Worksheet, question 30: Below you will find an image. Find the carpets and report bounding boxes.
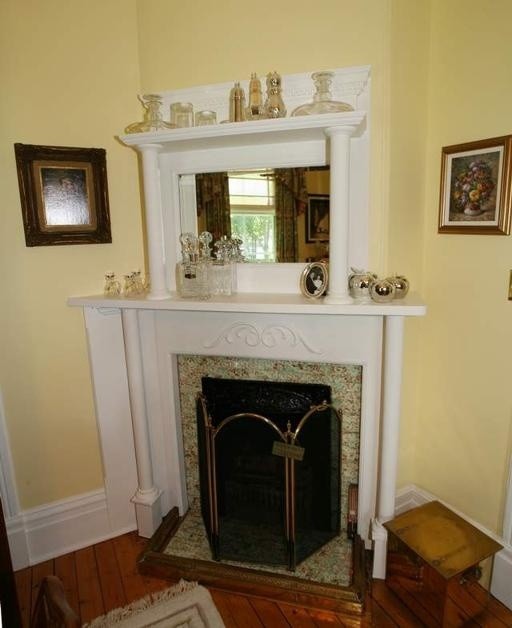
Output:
[71,577,227,627]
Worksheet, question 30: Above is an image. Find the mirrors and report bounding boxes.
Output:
[174,163,332,267]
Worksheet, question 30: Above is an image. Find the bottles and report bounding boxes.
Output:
[291,71,355,116]
[125,93,176,134]
[176,231,235,301]
[228,70,288,121]
[104,271,144,298]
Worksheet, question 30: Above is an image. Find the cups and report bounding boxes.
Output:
[170,102,217,128]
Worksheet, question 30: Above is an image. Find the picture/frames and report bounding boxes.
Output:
[298,257,331,300]
[12,142,114,248]
[434,134,512,237]
[303,191,330,246]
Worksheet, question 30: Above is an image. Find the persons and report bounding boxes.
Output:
[307,271,324,295]
